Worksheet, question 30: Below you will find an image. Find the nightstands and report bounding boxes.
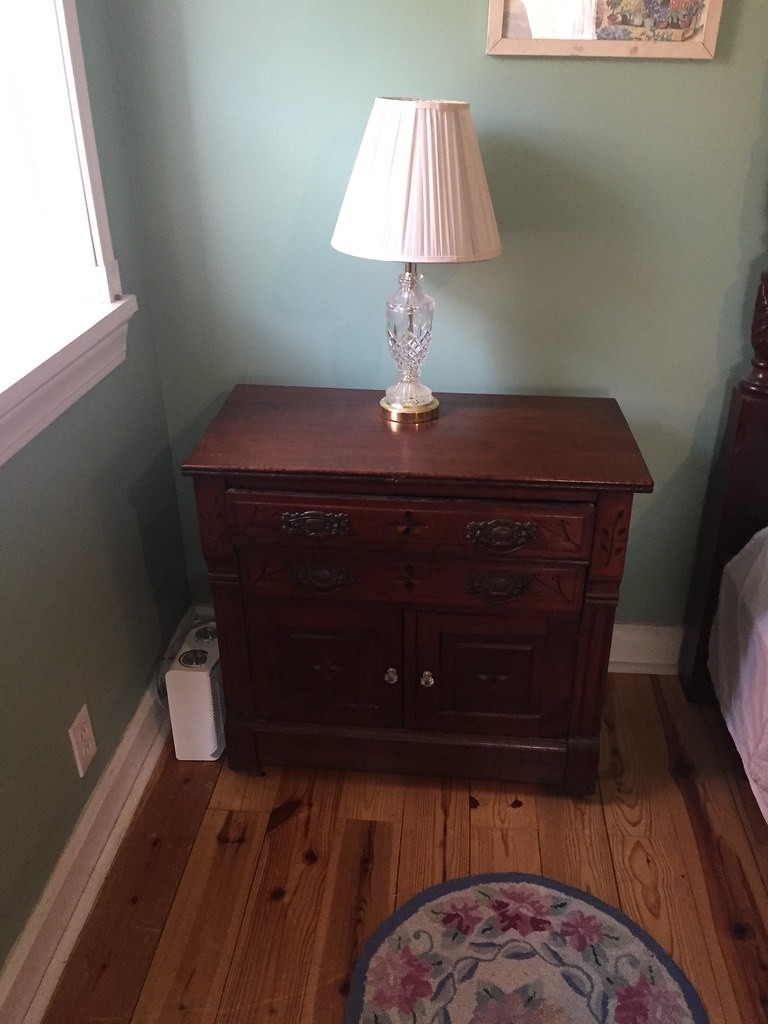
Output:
[184,388,656,801]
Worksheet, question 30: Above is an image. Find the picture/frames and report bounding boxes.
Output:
[485,0,723,60]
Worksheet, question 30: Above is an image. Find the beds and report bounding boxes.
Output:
[675,269,768,824]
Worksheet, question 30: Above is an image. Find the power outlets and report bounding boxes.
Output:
[68,702,97,778]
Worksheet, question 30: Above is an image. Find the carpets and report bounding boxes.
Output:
[344,872,710,1024]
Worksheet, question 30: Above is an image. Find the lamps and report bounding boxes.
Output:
[331,96,501,426]
